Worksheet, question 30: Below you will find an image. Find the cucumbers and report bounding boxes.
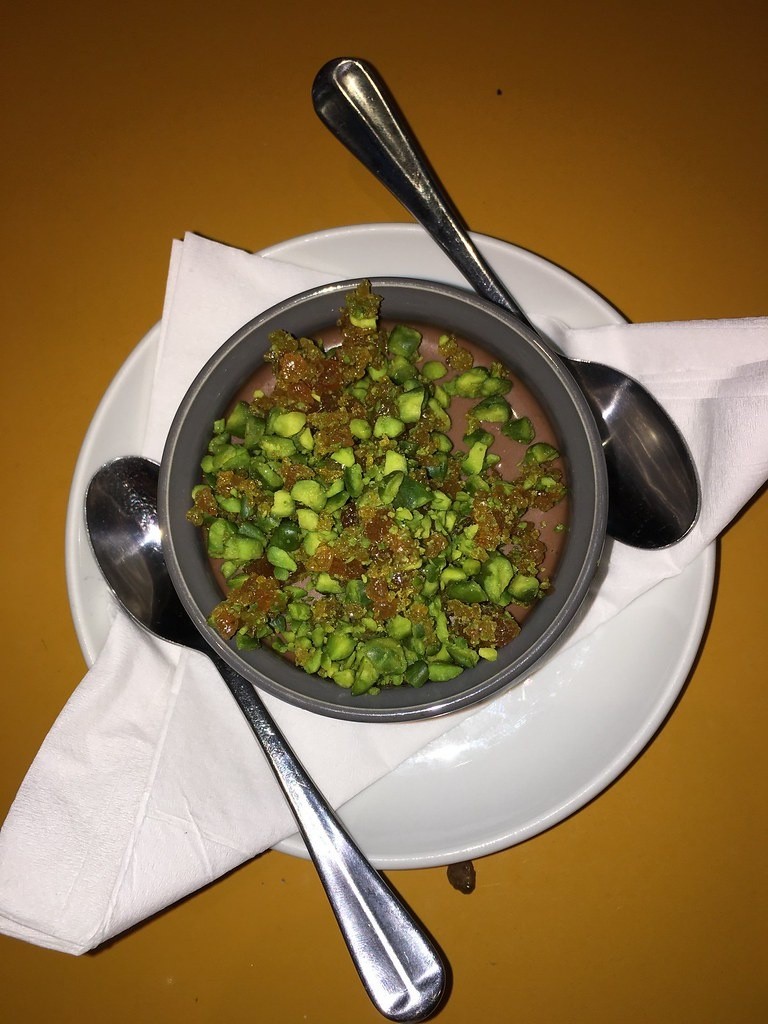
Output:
[191,308,560,692]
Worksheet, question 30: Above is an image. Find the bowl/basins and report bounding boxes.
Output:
[157,275,611,726]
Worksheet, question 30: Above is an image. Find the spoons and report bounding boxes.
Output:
[310,54,702,552]
[85,453,452,1024]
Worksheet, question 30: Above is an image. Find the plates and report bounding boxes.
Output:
[63,223,720,871]
